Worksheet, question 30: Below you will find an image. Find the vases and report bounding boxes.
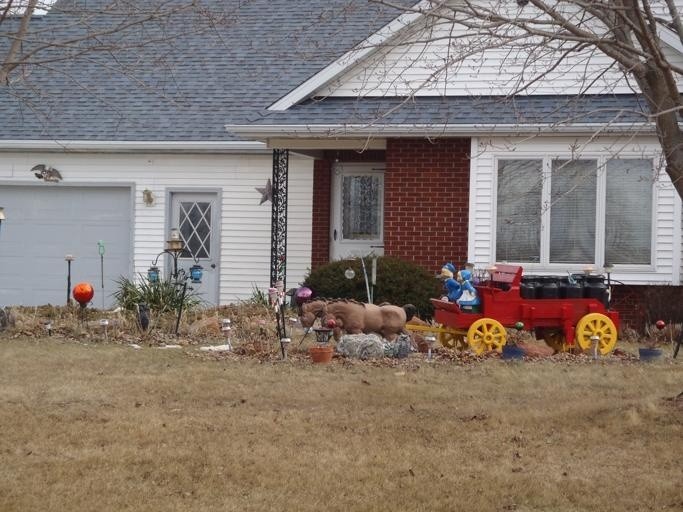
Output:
[310,347,332,364]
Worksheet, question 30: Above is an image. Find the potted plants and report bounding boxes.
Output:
[638,320,666,365]
